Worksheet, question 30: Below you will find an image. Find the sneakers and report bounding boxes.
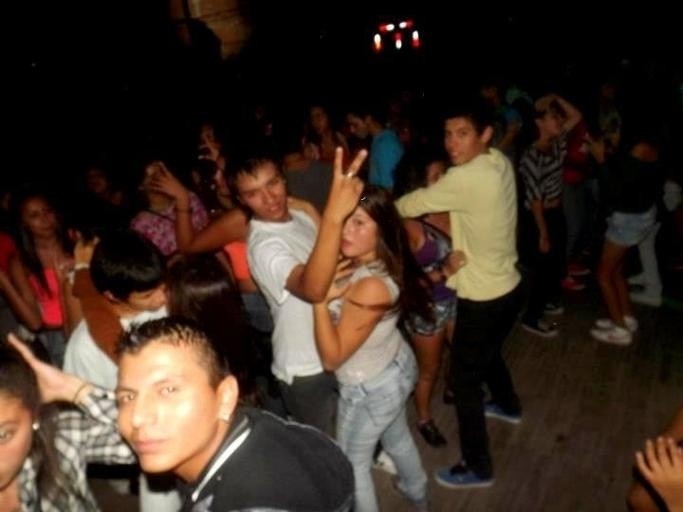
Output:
[432,461,497,492]
[441,388,466,409]
[416,419,450,446]
[483,397,527,427]
[516,268,668,346]
[372,444,432,512]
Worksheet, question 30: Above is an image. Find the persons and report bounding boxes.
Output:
[2,82,682,512]
